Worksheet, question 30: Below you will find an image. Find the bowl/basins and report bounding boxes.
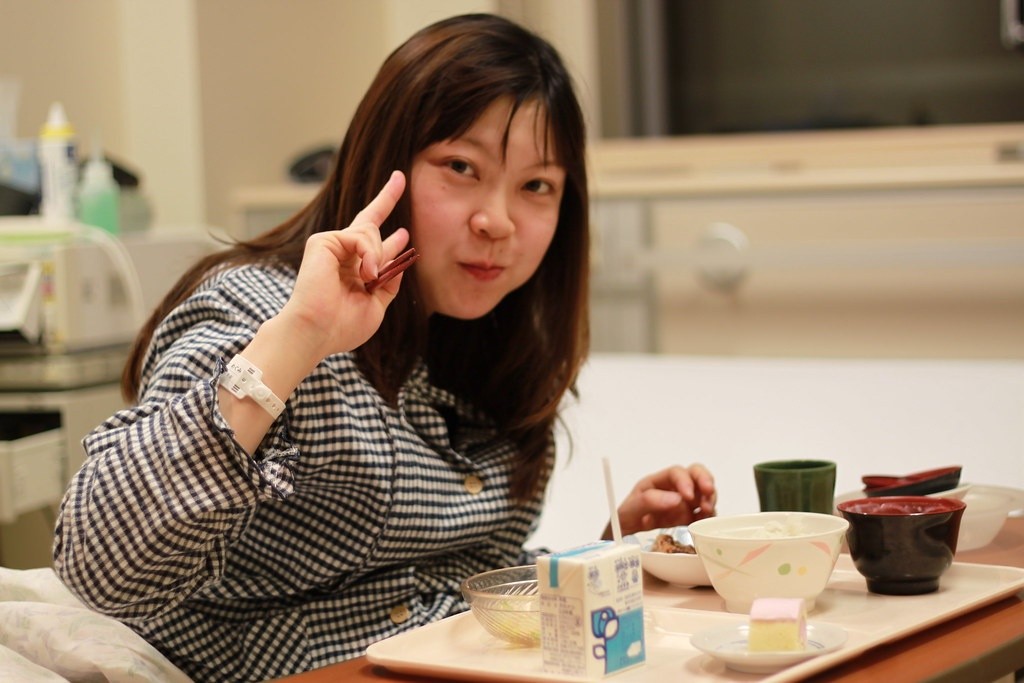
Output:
[838,466,1024,596]
[462,565,542,648]
[623,526,714,586]
[687,511,850,614]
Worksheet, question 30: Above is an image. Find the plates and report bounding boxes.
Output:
[691,623,848,673]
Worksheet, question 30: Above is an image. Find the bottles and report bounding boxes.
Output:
[37,104,77,229]
[78,150,120,237]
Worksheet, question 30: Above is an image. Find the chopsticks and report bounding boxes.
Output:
[365,248,419,293]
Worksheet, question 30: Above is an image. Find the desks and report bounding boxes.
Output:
[267,484,1024,683]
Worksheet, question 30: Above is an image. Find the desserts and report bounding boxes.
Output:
[746,596,807,652]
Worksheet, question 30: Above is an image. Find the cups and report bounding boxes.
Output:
[754,459,837,515]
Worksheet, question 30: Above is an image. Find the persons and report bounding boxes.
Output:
[53,15,717,683]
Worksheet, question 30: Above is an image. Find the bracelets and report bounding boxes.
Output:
[218,354,286,420]
